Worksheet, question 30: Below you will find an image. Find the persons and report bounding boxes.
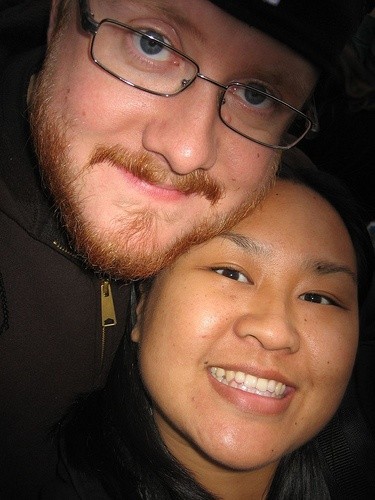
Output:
[0,0,375,500]
[46,146,375,500]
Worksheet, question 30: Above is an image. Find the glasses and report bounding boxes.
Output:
[74,0,320,152]
